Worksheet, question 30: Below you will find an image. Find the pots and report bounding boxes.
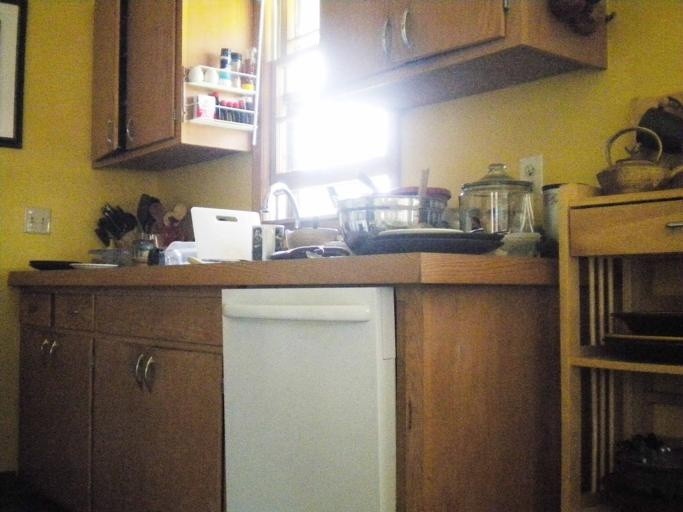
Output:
[612,431,683,502]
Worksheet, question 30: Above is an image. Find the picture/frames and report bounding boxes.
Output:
[0,0,29,149]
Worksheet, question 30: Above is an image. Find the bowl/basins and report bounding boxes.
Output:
[337,195,451,255]
[286,227,338,250]
[497,230,545,258]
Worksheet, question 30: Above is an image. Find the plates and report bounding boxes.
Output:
[269,246,349,257]
[29,260,119,270]
[610,310,683,336]
[601,332,683,366]
[349,227,505,255]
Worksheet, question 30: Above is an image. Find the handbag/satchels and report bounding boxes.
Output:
[636,105,683,152]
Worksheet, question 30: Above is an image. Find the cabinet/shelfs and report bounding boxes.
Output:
[17,287,94,512]
[556,183,683,510]
[93,290,221,512]
[90,0,261,170]
[320,1,609,110]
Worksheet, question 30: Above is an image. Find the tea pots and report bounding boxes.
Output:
[595,126,683,196]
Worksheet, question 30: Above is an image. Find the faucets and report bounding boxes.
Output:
[260,181,303,229]
